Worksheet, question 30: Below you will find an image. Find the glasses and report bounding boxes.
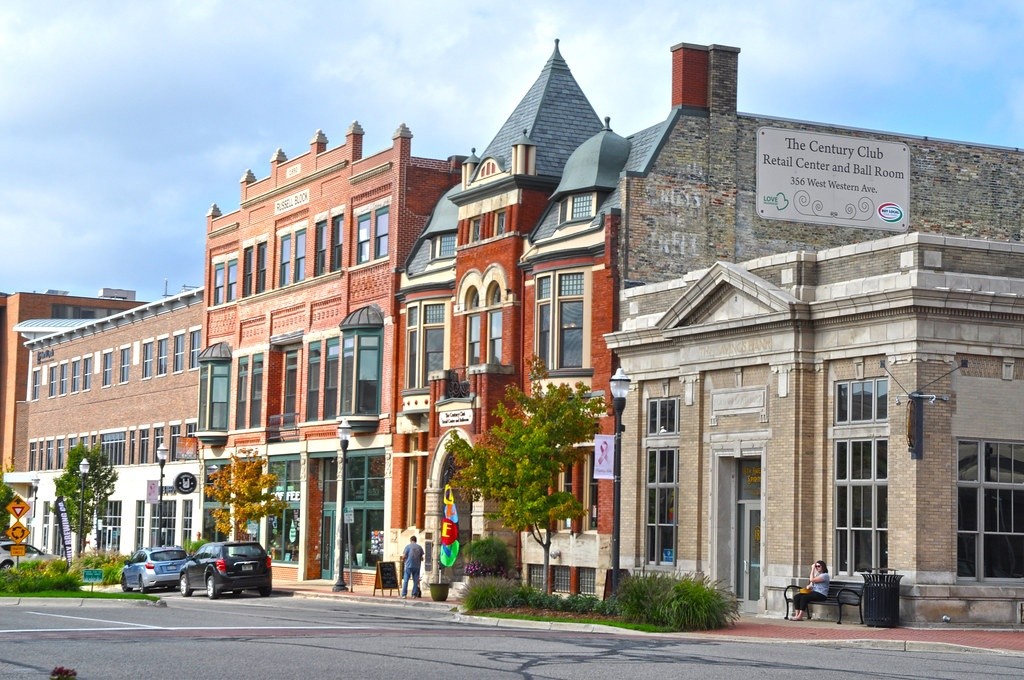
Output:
[815,565,821,567]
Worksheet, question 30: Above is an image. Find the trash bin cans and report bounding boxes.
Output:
[860,568,905,627]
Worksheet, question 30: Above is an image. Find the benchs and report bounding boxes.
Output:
[783,580,865,625]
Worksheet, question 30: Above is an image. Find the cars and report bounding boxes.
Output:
[0,542,57,571]
[119,546,192,593]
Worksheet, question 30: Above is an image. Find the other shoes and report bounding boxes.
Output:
[792,617,803,621]
[789,616,796,620]
[402,596,406,598]
[412,595,416,598]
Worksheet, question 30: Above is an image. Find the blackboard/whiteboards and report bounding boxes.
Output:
[378,562,399,589]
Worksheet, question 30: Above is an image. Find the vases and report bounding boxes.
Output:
[429,582,449,601]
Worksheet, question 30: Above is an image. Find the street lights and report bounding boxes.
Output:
[607,367,631,592]
[156,443,169,546]
[332,418,352,594]
[31,473,41,546]
[77,457,91,558]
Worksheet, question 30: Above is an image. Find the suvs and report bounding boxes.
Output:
[179,539,273,600]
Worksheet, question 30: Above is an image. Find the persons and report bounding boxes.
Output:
[789,560,829,621]
[400,536,424,599]
[197,532,201,540]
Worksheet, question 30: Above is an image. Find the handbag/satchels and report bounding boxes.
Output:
[799,588,812,594]
